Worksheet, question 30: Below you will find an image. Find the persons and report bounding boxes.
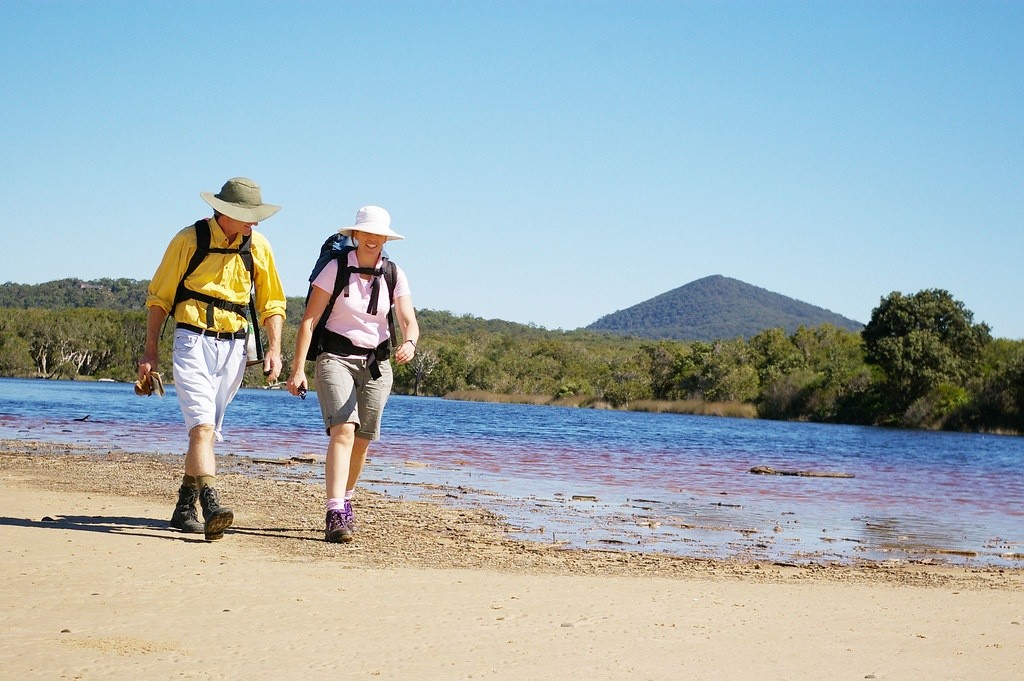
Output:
[286,205,420,541]
[136,176,287,541]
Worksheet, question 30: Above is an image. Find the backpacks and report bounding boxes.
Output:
[305,232,398,348]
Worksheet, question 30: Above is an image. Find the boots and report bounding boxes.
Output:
[198,483,234,541]
[168,484,205,533]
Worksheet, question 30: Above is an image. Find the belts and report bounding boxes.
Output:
[176,321,247,339]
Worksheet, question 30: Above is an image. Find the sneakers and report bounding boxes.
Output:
[324,508,353,543]
[343,499,359,531]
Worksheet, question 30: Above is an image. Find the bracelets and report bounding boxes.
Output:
[404,340,417,350]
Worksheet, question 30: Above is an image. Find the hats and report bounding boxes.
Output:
[338,206,405,242]
[200,177,281,223]
